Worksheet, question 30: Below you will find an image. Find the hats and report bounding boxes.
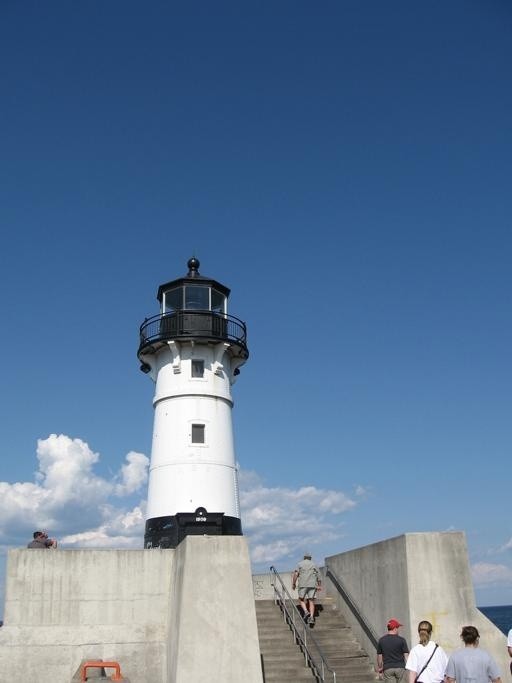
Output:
[387,619,403,627]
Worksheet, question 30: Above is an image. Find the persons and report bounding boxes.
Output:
[377,620,409,683]
[506,629,512,676]
[405,621,456,683]
[27,531,58,548]
[443,625,503,683]
[292,553,322,623]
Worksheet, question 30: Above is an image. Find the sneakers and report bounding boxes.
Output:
[302,611,311,620]
[307,616,315,623]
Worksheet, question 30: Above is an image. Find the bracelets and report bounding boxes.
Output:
[379,669,384,673]
